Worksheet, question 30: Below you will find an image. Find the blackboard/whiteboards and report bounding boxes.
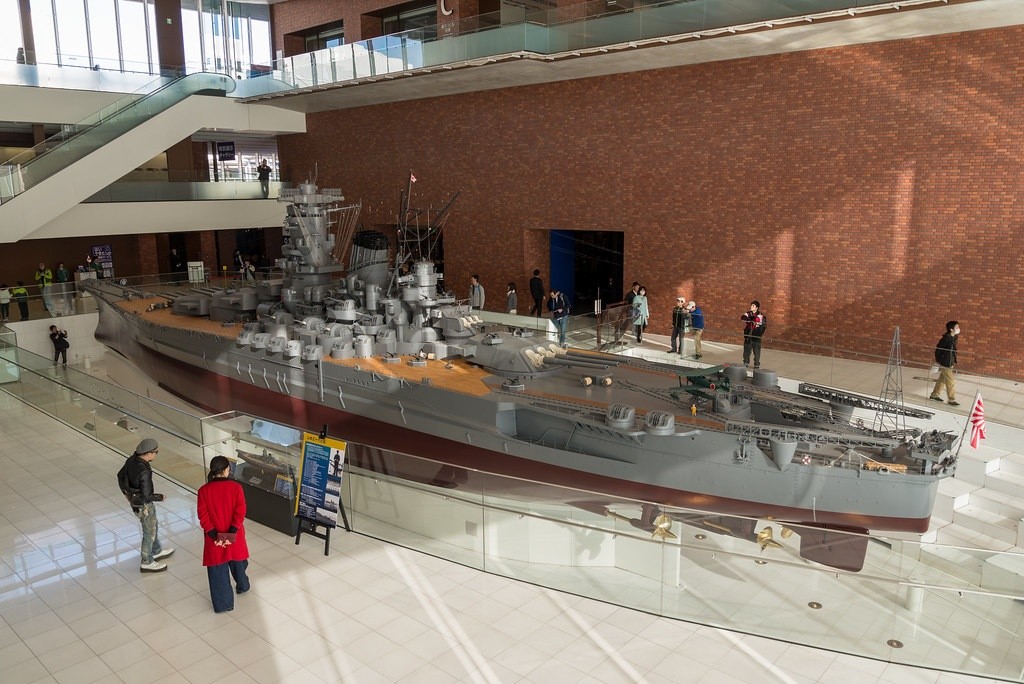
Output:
[187,261,205,283]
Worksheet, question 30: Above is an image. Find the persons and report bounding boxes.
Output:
[632,287,649,344]
[17,48,25,64]
[233,249,243,280]
[530,269,545,318]
[332,451,340,476]
[691,404,696,416]
[168,250,182,285]
[667,297,703,361]
[929,321,960,405]
[504,282,517,313]
[399,264,408,276]
[547,289,570,348]
[240,260,255,281]
[626,281,640,337]
[0,256,104,323]
[49,325,68,378]
[117,439,175,573]
[467,275,485,310]
[741,301,766,369]
[198,456,250,614]
[258,159,271,198]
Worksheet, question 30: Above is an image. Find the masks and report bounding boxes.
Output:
[954,328,960,334]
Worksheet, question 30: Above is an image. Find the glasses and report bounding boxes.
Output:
[149,450,159,454]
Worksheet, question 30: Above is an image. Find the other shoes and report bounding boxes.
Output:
[755,363,760,367]
[677,350,681,355]
[667,349,676,353]
[743,363,749,366]
[948,401,959,405]
[930,395,943,401]
[694,354,701,360]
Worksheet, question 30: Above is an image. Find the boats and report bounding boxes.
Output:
[77,159,974,577]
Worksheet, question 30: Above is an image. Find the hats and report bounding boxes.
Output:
[632,281,641,287]
[686,301,696,309]
[136,438,159,454]
[676,297,685,303]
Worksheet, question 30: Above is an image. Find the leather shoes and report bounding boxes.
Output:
[153,548,174,561]
[140,560,167,572]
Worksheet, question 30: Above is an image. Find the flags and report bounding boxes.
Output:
[969,397,986,449]
